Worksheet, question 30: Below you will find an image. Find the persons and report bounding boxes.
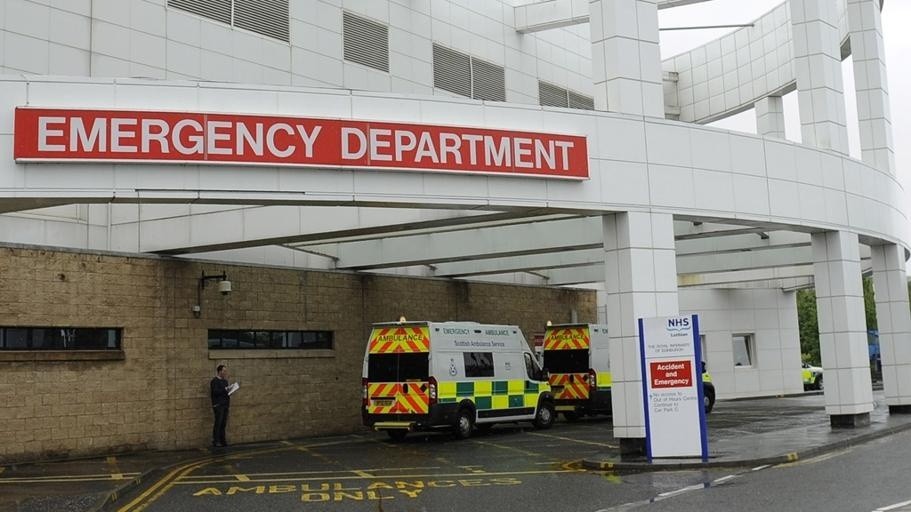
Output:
[211,365,231,447]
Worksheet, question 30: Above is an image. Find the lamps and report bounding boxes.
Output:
[201,267,231,296]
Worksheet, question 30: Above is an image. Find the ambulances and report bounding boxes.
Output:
[542,322,716,420]
[362,316,556,442]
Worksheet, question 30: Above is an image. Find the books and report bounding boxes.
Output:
[228,383,240,396]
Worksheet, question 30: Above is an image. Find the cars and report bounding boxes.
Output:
[802,362,824,390]
